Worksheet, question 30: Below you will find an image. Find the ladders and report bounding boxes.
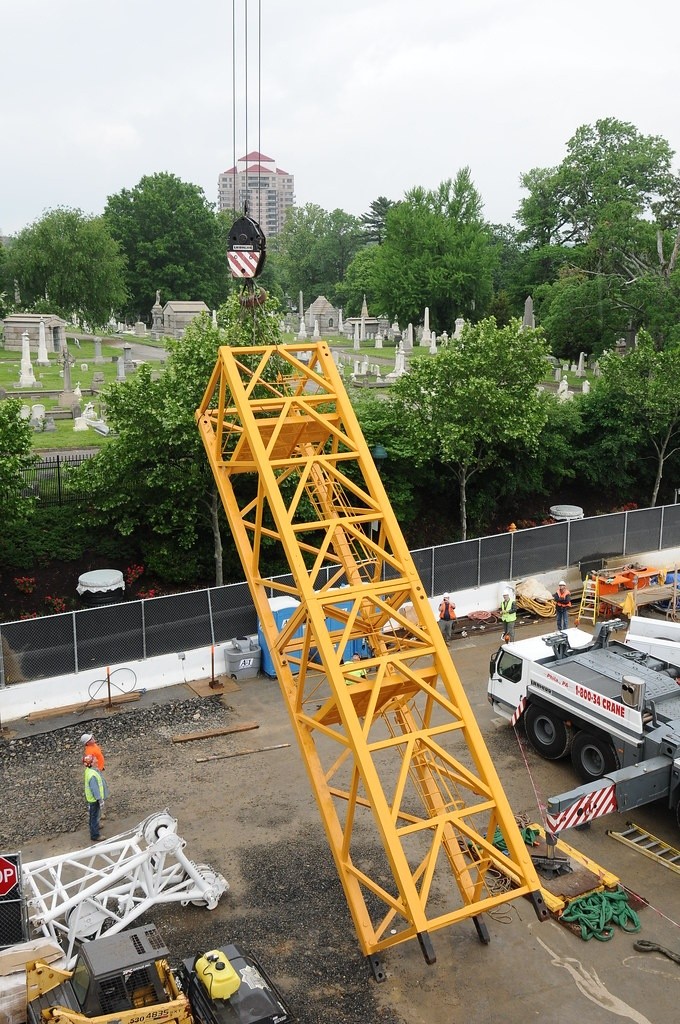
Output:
[576,575,599,627]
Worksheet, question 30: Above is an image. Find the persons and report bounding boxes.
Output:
[344,652,366,687]
[555,581,571,630]
[81,734,105,772]
[84,755,105,841]
[438,592,455,647]
[499,592,517,643]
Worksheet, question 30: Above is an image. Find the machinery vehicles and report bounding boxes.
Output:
[24,922,292,1024]
[485,619,680,825]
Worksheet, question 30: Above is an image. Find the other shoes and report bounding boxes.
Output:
[446,641,450,646]
[100,812,107,820]
[93,835,106,841]
[99,823,104,828]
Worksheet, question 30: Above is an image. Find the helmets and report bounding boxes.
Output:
[81,734,92,745]
[559,581,566,585]
[82,754,93,767]
[443,593,449,597]
[503,590,509,595]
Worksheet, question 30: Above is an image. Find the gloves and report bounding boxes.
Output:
[500,610,507,615]
[98,799,105,810]
